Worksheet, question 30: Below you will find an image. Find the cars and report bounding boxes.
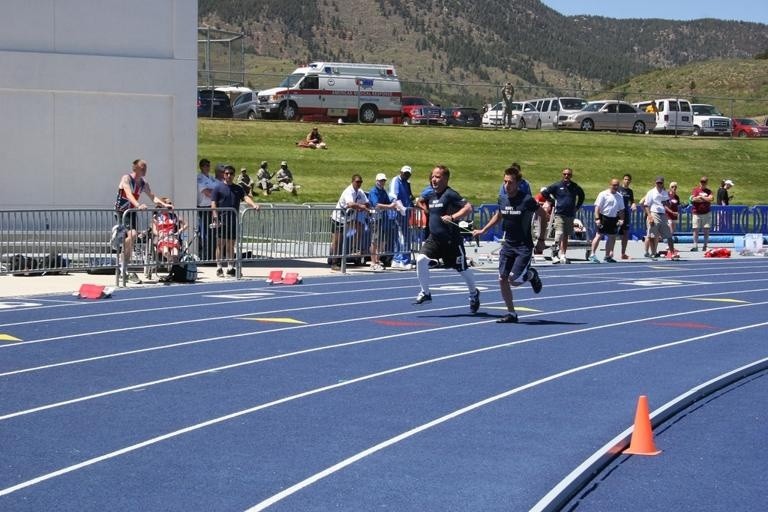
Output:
[550,100,658,134]
[729,118,768,139]
[479,101,543,130]
[231,89,263,120]
[440,107,482,128]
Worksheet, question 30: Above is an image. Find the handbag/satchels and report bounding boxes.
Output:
[87,257,117,274]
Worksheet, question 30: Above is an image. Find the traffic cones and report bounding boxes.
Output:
[620,394,667,458]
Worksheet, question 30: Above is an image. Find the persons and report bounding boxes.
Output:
[471,167,547,323]
[151,198,188,263]
[197,159,225,265]
[500,162,532,195]
[326,165,440,276]
[587,174,680,264]
[501,82,514,130]
[306,127,322,148]
[690,176,714,251]
[645,101,659,134]
[210,165,260,276]
[276,160,297,194]
[237,168,254,198]
[534,187,555,221]
[717,180,735,231]
[410,165,480,313]
[546,168,585,264]
[256,161,279,195]
[114,159,175,284]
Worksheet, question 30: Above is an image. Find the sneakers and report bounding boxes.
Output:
[496,313,518,323]
[370,262,385,271]
[604,256,616,262]
[530,268,542,294]
[552,256,560,263]
[226,268,242,277]
[416,291,432,301]
[119,273,128,282]
[588,255,600,263]
[690,247,698,252]
[391,261,415,270]
[331,263,340,270]
[469,288,479,313]
[217,268,224,277]
[128,273,142,284]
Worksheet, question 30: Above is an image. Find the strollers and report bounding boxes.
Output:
[140,204,187,278]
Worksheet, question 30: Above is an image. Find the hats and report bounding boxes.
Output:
[241,168,246,171]
[281,160,288,166]
[656,176,664,183]
[400,166,412,174]
[725,180,734,186]
[376,173,387,181]
[700,176,708,182]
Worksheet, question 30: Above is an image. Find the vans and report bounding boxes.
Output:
[629,98,695,136]
[528,97,589,132]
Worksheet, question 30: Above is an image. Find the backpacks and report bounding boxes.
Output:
[174,251,197,281]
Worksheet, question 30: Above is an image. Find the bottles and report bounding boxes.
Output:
[209,222,223,229]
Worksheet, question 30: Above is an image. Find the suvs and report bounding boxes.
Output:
[691,103,733,137]
[197,88,233,119]
[394,96,442,126]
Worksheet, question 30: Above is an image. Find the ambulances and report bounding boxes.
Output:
[255,61,403,124]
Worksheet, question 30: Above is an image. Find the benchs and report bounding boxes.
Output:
[494,233,592,260]
